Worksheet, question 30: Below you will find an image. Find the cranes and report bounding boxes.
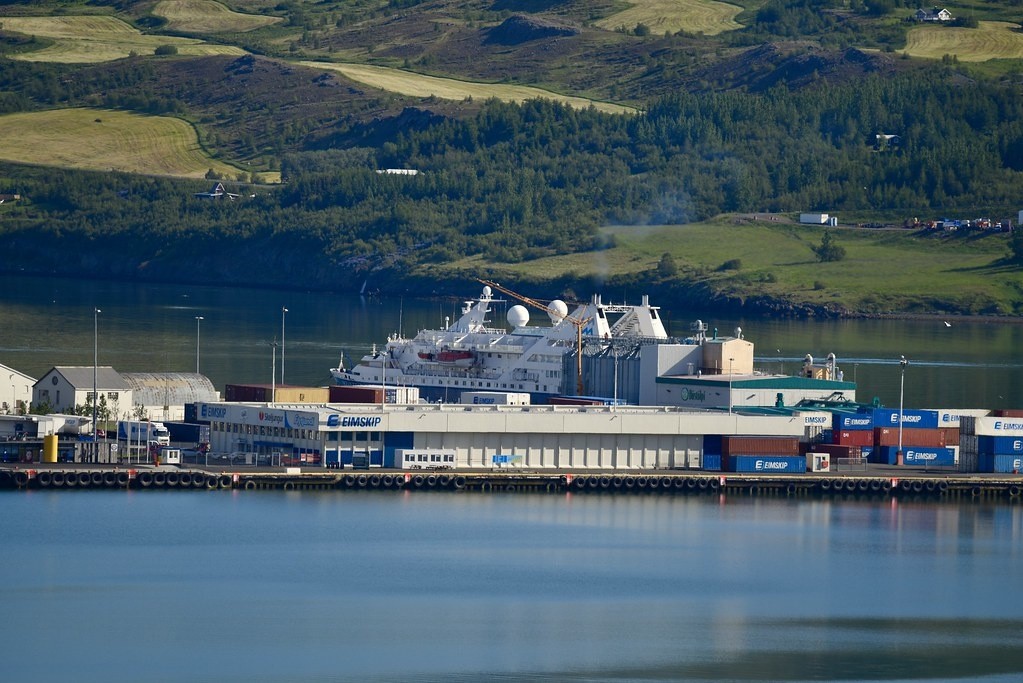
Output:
[473,276,594,393]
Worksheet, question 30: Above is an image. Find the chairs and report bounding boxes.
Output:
[409,464,452,471]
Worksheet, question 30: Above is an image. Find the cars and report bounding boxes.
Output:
[275,457,301,466]
[211,452,270,462]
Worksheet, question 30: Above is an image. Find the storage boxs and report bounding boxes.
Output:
[703,405,1023,476]
[225,382,531,405]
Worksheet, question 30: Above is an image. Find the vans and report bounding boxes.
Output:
[180,448,202,457]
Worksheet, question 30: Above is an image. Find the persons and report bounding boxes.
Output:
[22,450,33,463]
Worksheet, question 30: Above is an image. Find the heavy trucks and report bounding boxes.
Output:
[118,421,169,446]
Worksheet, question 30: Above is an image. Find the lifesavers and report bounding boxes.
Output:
[219,476,232,488]
[78,473,91,487]
[205,477,218,488]
[15,473,29,486]
[117,473,130,486]
[154,473,166,486]
[193,474,205,487]
[140,472,153,487]
[179,474,192,486]
[52,472,64,486]
[283,481,295,491]
[166,473,179,486]
[104,473,117,486]
[357,475,1021,498]
[92,473,103,485]
[244,480,256,490]
[346,475,355,487]
[65,473,78,486]
[38,473,51,486]
[4,473,12,484]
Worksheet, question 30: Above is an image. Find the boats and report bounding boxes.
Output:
[327,286,671,403]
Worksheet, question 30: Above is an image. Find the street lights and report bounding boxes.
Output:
[897,355,908,465]
[195,316,204,373]
[93,306,101,441]
[283,305,289,384]
